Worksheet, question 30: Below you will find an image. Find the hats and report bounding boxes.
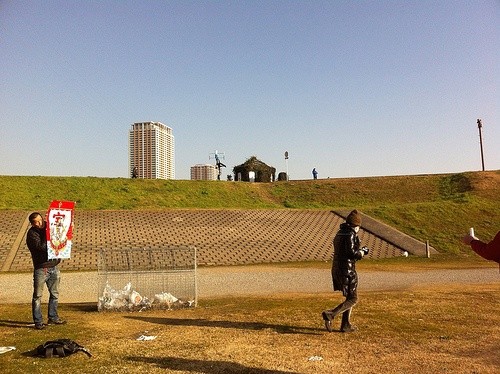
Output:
[346,209,362,226]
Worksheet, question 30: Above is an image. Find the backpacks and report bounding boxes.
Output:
[38,339,79,358]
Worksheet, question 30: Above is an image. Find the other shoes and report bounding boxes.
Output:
[35,322,45,330]
[322,310,334,332]
[340,322,355,332]
[47,318,67,325]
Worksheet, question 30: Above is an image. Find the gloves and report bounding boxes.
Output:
[362,246,369,255]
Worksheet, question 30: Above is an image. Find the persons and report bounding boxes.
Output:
[132,168,138,178]
[322,210,369,333]
[26,212,74,329]
[464,231,500,268]
[313,168,318,179]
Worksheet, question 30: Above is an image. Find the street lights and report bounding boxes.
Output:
[477,118,486,171]
